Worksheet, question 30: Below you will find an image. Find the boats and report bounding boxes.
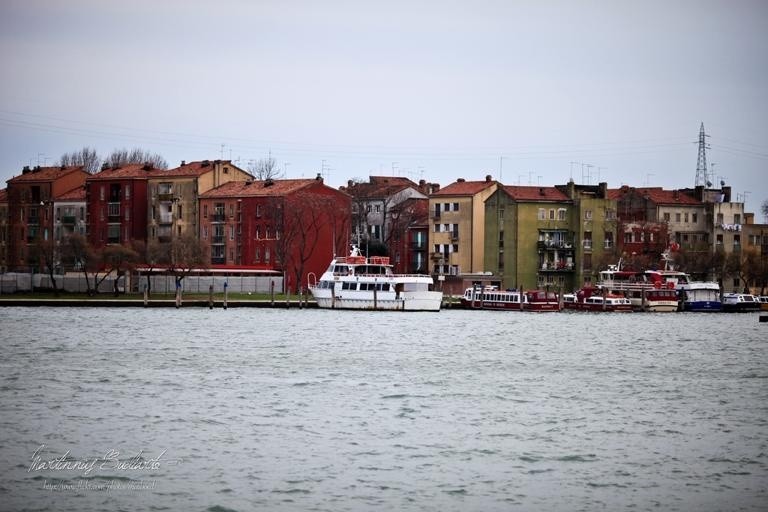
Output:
[307,256,443,310]
